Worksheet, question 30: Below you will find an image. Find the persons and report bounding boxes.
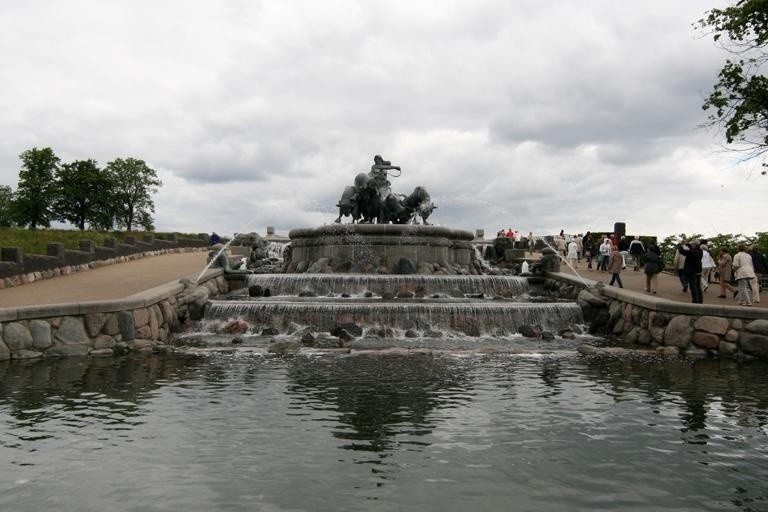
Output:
[209,232,220,247]
[496,228,521,249]
[555,230,767,306]
[350,155,392,211]
[527,232,537,255]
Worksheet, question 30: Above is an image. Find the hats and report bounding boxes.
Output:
[634,234,640,239]
[689,239,700,246]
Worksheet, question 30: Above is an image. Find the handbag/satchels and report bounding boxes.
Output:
[645,261,664,274]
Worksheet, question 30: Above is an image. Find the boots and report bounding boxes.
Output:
[588,266,639,272]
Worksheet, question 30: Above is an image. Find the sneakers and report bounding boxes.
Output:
[703,284,708,291]
[733,290,739,298]
[683,285,688,293]
[644,287,651,292]
[652,289,657,294]
[716,294,727,298]
[737,299,760,306]
